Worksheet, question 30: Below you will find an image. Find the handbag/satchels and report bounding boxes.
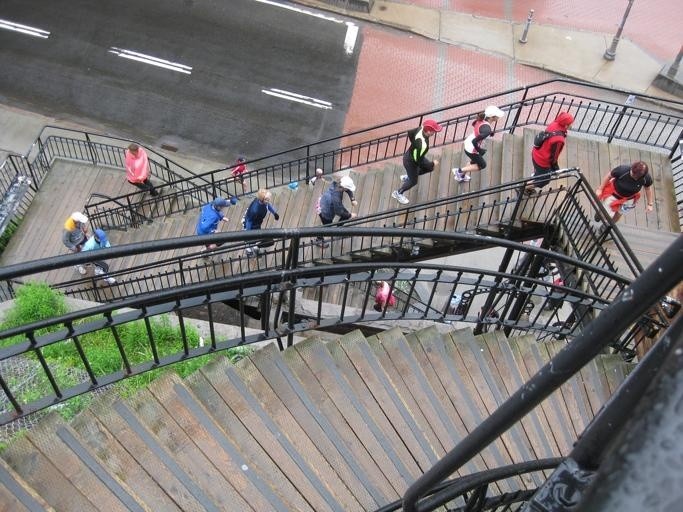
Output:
[533,131,566,149]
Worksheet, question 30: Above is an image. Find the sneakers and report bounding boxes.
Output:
[515,183,537,196]
[454,176,472,182]
[392,190,409,204]
[311,237,330,249]
[253,246,264,258]
[400,174,409,182]
[94,268,109,278]
[73,265,88,275]
[245,245,253,257]
[595,211,601,223]
[451,167,464,183]
[104,277,118,286]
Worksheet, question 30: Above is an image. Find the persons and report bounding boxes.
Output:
[516,111,574,195]
[62,212,89,252]
[594,161,653,233]
[124,144,158,196]
[313,176,358,248]
[231,158,247,184]
[391,120,442,204]
[240,189,279,254]
[197,197,229,251]
[79,229,116,284]
[634,280,683,362]
[373,281,390,312]
[451,105,505,183]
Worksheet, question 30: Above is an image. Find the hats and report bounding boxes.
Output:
[341,176,357,192]
[422,119,443,132]
[484,106,505,118]
[288,179,299,190]
[70,211,89,224]
[236,155,248,163]
[214,197,230,208]
[94,228,108,243]
